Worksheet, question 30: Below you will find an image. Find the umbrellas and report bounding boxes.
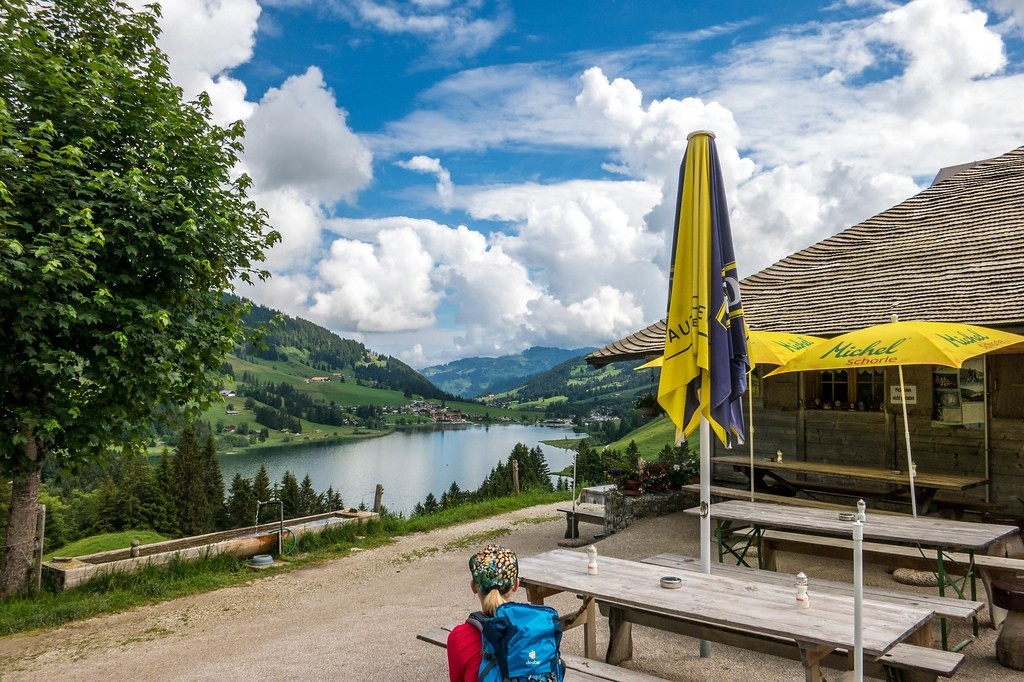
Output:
[636,322,1024,519]
[657,128,752,663]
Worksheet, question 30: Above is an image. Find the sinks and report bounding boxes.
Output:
[42,509,381,591]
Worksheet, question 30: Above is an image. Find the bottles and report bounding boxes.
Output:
[777,450,783,463]
[587,544,598,574]
[795,571,810,608]
[912,461,917,477]
[857,499,866,522]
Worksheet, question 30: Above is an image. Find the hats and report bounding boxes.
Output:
[469,544,520,596]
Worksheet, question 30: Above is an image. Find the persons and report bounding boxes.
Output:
[447,544,520,682]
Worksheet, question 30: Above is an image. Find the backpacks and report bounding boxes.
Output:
[469,601,566,682]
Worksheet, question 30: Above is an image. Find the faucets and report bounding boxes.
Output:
[256,500,267,507]
